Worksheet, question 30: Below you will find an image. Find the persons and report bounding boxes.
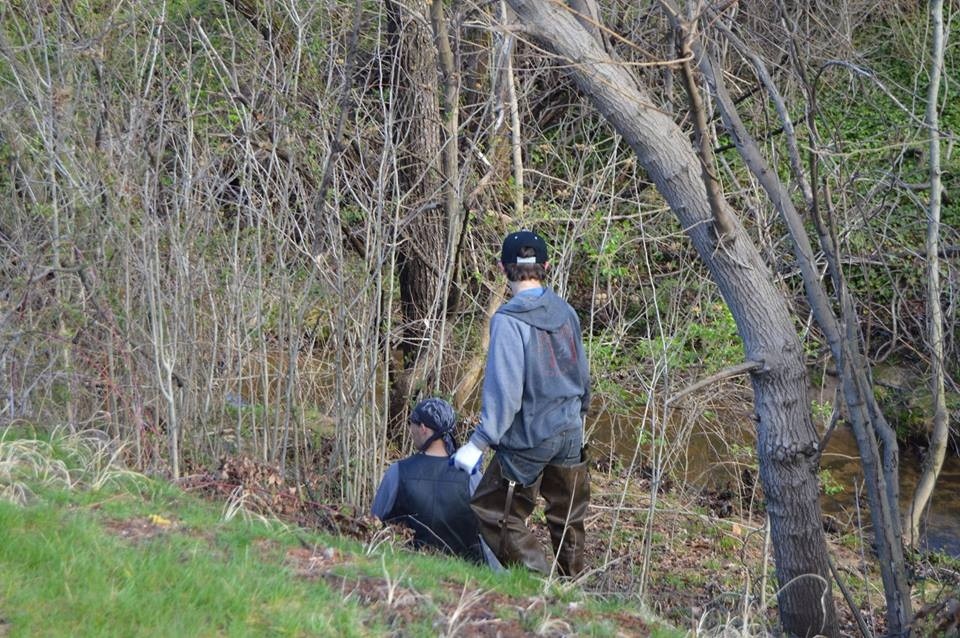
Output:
[454,232,590,586]
[371,398,503,569]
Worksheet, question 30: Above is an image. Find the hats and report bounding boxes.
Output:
[501,232,547,263]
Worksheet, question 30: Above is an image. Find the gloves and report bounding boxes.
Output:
[449,441,483,476]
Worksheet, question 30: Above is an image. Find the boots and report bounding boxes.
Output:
[473,454,548,579]
[540,448,592,583]
